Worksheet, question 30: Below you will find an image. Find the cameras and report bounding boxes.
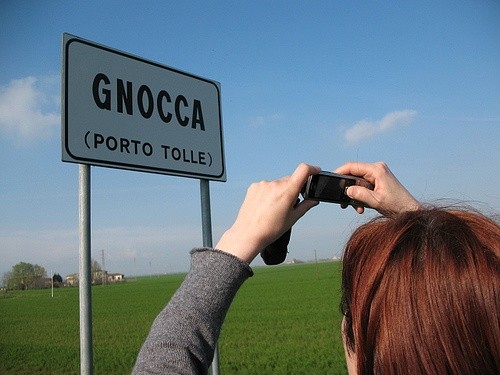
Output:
[300,170,375,208]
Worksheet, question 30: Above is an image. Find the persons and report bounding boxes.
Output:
[134,165,500,375]
